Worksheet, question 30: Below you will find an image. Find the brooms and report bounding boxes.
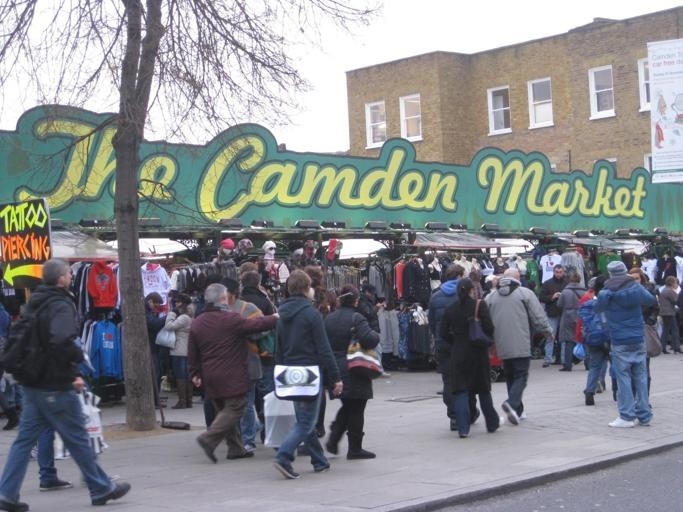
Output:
[147,336,190,429]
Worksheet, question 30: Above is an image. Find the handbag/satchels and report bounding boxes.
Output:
[273,364,320,400]
[643,322,662,356]
[155,327,176,350]
[346,335,382,380]
[467,317,494,347]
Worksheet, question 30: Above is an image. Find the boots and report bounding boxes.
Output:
[172,379,192,409]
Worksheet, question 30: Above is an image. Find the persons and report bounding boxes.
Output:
[439,278,499,438]
[216,239,307,278]
[417,256,529,285]
[484,268,555,426]
[428,264,480,431]
[145,261,392,480]
[0,258,131,512]
[37,427,74,492]
[539,259,683,429]
[0,304,24,430]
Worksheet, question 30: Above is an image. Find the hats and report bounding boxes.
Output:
[606,260,626,278]
[175,294,191,305]
[503,268,520,281]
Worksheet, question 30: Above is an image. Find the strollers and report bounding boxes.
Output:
[487,346,504,382]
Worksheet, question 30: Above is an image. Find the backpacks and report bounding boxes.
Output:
[578,302,610,346]
[0,297,76,387]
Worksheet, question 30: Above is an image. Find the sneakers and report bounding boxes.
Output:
[0,499,29,512]
[39,480,71,491]
[92,482,130,505]
[596,380,603,393]
[542,357,573,371]
[585,391,594,405]
[275,421,376,479]
[197,436,217,463]
[450,401,526,437]
[228,443,256,460]
[608,418,635,427]
